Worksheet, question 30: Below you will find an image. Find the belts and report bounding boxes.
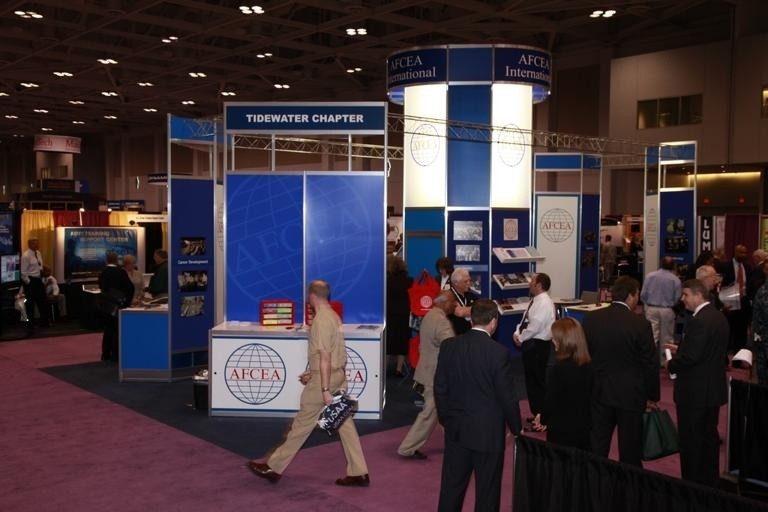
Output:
[648,304,672,308]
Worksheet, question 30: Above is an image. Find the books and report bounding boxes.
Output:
[494,245,544,315]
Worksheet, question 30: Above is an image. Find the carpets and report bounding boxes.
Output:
[34,357,525,464]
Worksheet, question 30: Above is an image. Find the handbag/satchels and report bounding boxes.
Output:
[318,392,356,436]
[640,404,681,461]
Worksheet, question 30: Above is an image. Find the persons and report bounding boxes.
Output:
[120,255,146,305]
[395,288,458,461]
[14,285,30,322]
[432,256,456,287]
[629,232,642,276]
[598,234,619,283]
[529,316,595,461]
[387,252,412,378]
[178,238,208,316]
[663,278,731,486]
[579,276,660,470]
[19,235,52,328]
[513,272,558,433]
[432,296,522,511]
[446,268,480,335]
[639,244,768,447]
[98,251,133,360]
[245,278,372,489]
[140,249,170,302]
[39,265,66,320]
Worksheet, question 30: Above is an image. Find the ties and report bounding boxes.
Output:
[35,250,41,266]
[737,264,744,297]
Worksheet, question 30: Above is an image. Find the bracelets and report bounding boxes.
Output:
[320,383,331,394]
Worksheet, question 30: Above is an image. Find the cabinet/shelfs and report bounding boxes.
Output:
[492,247,546,316]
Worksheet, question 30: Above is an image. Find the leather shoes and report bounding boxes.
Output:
[335,474,369,486]
[409,451,428,459]
[247,460,281,483]
